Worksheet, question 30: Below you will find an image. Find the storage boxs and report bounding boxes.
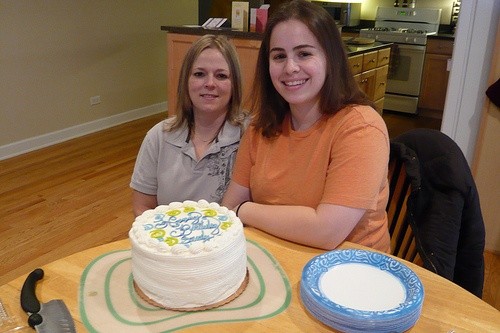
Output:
[232,2,271,31]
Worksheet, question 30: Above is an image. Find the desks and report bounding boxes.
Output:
[0,226,500,333]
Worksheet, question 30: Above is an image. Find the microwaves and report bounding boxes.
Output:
[310,1,351,26]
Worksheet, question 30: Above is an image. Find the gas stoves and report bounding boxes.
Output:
[359,5,443,43]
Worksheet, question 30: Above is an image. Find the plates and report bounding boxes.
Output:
[300,248,424,333]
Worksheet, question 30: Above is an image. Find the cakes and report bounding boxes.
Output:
[128,199,247,309]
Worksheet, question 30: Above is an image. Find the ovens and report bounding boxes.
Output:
[357,28,427,115]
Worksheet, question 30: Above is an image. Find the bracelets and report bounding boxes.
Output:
[236,200,252,227]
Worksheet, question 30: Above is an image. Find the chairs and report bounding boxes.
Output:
[386,130,462,267]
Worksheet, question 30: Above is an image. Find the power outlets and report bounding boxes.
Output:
[90,96,100,105]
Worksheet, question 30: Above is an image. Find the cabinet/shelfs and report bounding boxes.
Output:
[418,39,454,119]
[348,47,390,117]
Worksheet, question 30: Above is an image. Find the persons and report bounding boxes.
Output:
[129,34,252,219]
[220,0,391,257]
